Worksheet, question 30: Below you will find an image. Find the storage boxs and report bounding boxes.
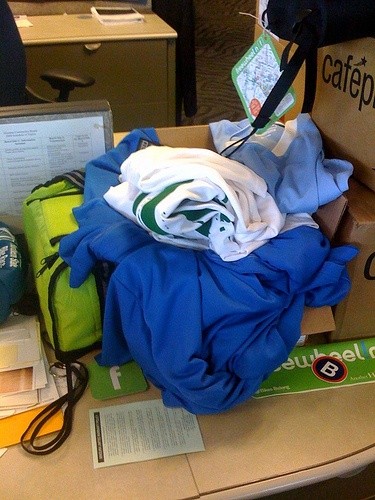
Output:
[256,25,375,193]
[113,125,347,338]
[321,177,375,342]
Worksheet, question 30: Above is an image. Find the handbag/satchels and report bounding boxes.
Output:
[22,171,107,360]
[260,0,374,49]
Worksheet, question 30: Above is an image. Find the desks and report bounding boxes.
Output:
[14,10,177,132]
[1,348,375,500]
